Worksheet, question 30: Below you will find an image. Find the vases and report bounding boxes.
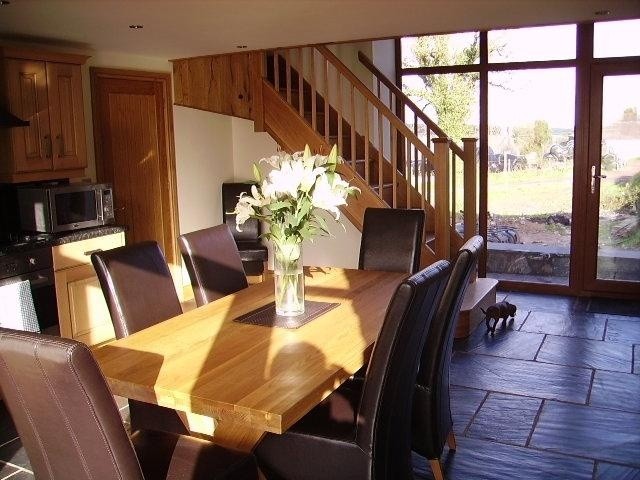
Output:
[273,241,305,317]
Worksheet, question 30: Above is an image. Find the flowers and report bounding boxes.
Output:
[226,144,361,309]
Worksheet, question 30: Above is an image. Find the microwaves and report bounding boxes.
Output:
[21,177,115,234]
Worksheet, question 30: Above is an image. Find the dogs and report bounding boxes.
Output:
[479,301,517,336]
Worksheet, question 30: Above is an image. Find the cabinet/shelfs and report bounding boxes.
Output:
[52,227,126,348]
[0,45,91,182]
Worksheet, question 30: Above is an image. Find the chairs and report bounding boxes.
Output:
[91,239,183,340]
[222,182,268,284]
[177,224,249,309]
[358,207,424,273]
[253,259,451,480]
[0,326,259,480]
[349,235,484,480]
